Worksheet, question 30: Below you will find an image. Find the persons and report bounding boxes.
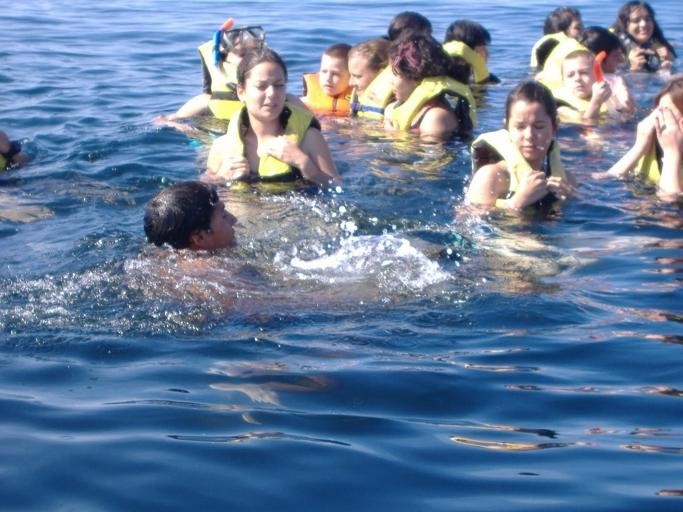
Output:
[0,130,136,224]
[127,182,548,317]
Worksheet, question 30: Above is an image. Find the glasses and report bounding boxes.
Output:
[219,27,264,52]
